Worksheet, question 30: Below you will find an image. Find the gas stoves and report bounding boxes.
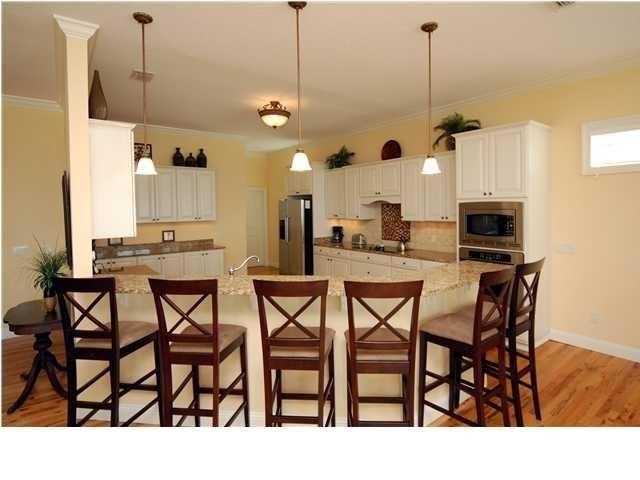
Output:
[366,246,415,252]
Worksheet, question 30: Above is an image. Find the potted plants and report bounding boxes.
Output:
[433,112,483,152]
[24,234,68,312]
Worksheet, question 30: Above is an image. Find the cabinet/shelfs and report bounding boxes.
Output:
[358,160,399,207]
[134,167,177,222]
[401,156,425,221]
[179,168,216,220]
[351,253,390,273]
[183,248,223,275]
[323,167,345,218]
[344,166,375,220]
[418,257,447,272]
[136,251,182,276]
[314,246,350,276]
[450,119,554,197]
[391,257,420,272]
[284,161,332,195]
[425,153,455,224]
[96,256,138,273]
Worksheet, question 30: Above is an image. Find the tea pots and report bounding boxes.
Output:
[398,236,406,251]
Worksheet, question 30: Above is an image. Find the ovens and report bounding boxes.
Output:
[459,247,524,307]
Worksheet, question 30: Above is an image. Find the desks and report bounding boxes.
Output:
[4,295,70,417]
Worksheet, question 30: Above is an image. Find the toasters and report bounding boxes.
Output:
[352,233,367,245]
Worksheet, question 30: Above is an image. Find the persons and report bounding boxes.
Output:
[134,145,150,158]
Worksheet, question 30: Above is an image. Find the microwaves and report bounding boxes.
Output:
[459,202,524,251]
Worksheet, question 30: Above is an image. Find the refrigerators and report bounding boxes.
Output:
[278,198,313,275]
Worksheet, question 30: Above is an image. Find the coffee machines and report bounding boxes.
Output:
[332,226,344,243]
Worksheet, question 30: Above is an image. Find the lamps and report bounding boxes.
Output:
[288,3,313,177]
[422,24,442,175]
[135,10,160,178]
[254,100,291,131]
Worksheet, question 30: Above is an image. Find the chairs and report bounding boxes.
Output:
[149,276,249,427]
[50,272,162,427]
[344,279,421,427]
[453,258,549,427]
[252,276,335,427]
[418,264,513,427]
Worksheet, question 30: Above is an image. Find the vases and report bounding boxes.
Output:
[171,145,208,168]
